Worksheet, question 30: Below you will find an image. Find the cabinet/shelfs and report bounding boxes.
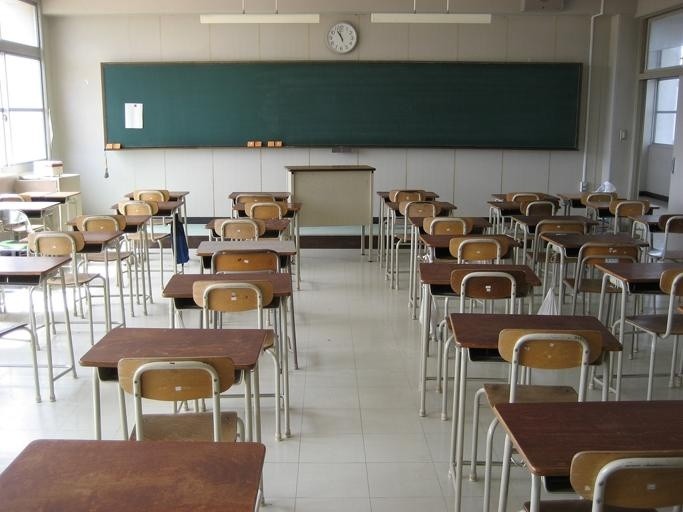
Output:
[14,173,82,230]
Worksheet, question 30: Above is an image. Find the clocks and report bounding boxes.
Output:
[324,21,358,55]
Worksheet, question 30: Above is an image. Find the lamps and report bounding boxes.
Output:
[370,0,491,24]
[200,0,320,24]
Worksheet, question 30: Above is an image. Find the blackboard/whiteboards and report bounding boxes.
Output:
[100,59,584,152]
